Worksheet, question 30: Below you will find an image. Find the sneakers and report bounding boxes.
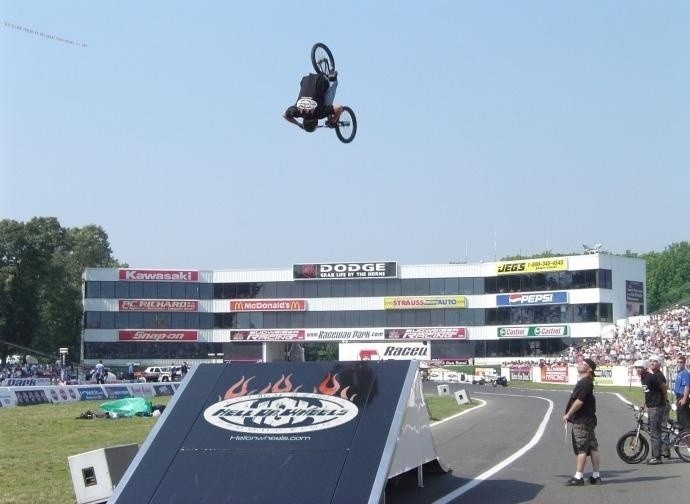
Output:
[647,458,663,465]
[660,453,670,459]
[563,476,584,486]
[587,476,602,485]
[328,71,337,81]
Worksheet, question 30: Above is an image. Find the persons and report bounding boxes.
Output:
[0,352,95,380]
[283,72,344,132]
[128,363,135,379]
[633,358,667,465]
[648,354,671,460]
[501,301,690,369]
[170,363,176,381]
[562,358,602,486]
[95,360,105,384]
[674,356,690,430]
[180,362,188,378]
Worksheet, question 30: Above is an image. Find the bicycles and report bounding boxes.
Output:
[311,42,358,144]
[616,403,690,464]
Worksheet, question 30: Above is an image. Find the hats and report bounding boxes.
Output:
[583,358,597,377]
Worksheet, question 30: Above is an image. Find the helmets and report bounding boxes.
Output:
[649,355,664,368]
[633,360,650,372]
[303,119,318,131]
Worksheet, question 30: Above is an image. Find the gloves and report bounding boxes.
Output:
[326,120,337,128]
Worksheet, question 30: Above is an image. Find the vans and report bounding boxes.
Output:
[143,366,191,382]
